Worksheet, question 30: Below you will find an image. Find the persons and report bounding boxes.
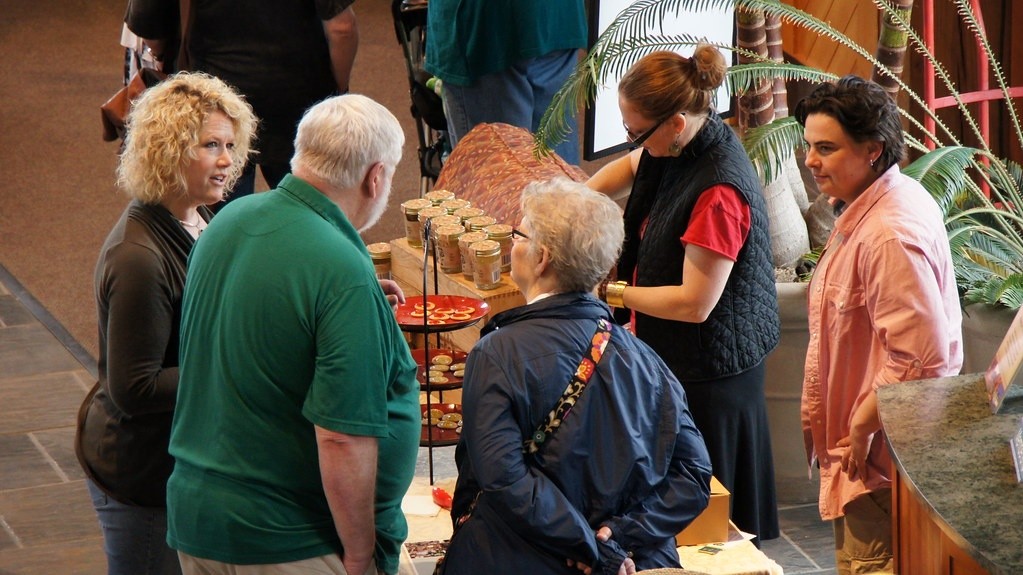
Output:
[74,69,265,575]
[120,0,359,217]
[794,73,965,575]
[166,92,421,575]
[582,42,781,552]
[423,0,588,165]
[430,174,713,575]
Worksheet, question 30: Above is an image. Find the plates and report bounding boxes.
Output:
[396,295,491,333]
[410,350,469,391]
[419,404,462,447]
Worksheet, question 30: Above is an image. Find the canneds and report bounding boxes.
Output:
[402,189,516,290]
[366,242,392,279]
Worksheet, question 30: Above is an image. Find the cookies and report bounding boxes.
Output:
[410,301,474,326]
[422,354,466,383]
[422,408,462,429]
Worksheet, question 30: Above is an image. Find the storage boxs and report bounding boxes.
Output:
[389,237,526,355]
[675,473,731,546]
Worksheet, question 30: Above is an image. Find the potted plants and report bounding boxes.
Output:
[534,0,1023,502]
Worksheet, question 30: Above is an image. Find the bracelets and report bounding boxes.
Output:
[599,279,629,309]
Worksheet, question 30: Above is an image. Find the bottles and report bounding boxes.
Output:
[142,45,163,71]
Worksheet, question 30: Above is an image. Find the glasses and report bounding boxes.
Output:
[512,226,531,243]
[621,113,688,149]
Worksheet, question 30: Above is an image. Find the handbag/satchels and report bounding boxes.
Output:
[101,65,167,141]
